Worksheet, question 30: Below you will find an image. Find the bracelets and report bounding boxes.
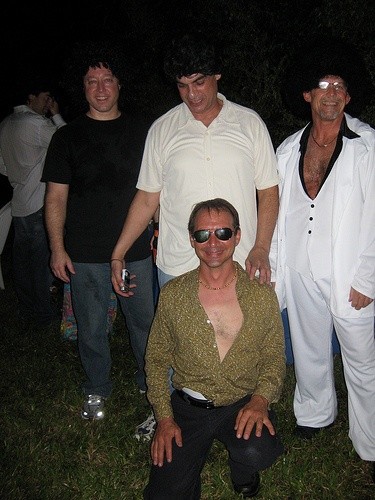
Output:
[110,259,125,264]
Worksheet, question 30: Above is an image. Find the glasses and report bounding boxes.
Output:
[317,81,349,93]
[192,227,235,243]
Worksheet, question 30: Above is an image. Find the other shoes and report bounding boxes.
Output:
[292,422,334,441]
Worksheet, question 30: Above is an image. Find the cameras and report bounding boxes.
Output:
[121,268,131,293]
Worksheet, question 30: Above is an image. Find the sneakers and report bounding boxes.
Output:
[131,410,159,444]
[80,394,106,420]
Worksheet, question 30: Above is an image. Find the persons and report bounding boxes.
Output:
[145,197,287,500]
[269,72,375,464]
[39,58,155,422]
[110,34,280,442]
[0,92,68,328]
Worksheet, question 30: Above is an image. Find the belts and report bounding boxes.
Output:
[174,389,224,410]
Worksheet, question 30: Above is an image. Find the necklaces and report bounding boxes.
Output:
[311,128,338,148]
[197,261,238,291]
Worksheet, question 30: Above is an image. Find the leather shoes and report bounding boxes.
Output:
[234,469,260,498]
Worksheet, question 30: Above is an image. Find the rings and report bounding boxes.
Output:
[360,297,365,300]
